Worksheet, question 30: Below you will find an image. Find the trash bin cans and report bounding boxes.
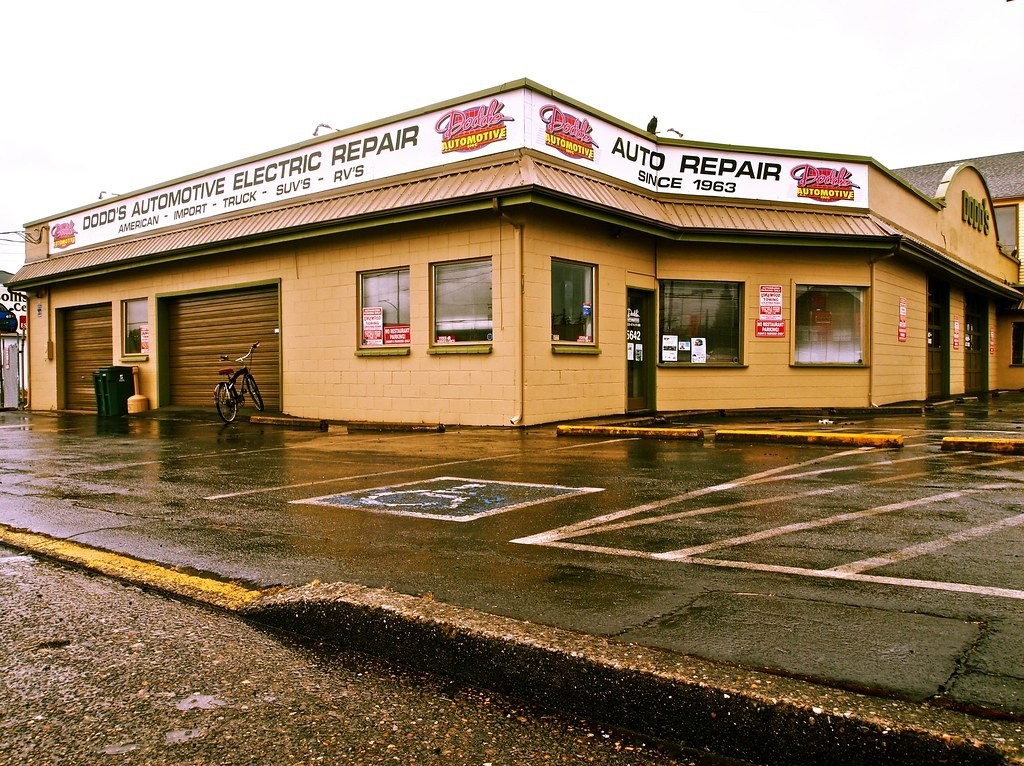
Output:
[92,366,135,417]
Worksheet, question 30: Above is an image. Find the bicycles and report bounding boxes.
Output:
[212,341,264,423]
